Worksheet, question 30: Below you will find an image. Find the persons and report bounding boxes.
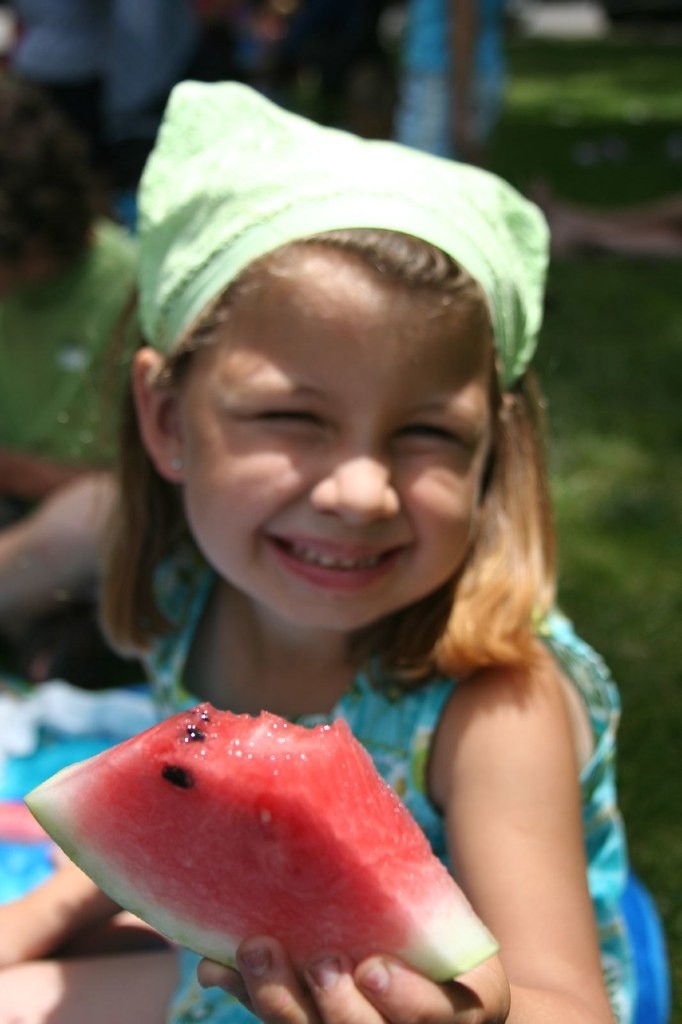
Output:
[0,80,639,1024]
[0,0,500,311]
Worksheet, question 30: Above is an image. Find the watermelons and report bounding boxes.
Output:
[23,702,499,982]
